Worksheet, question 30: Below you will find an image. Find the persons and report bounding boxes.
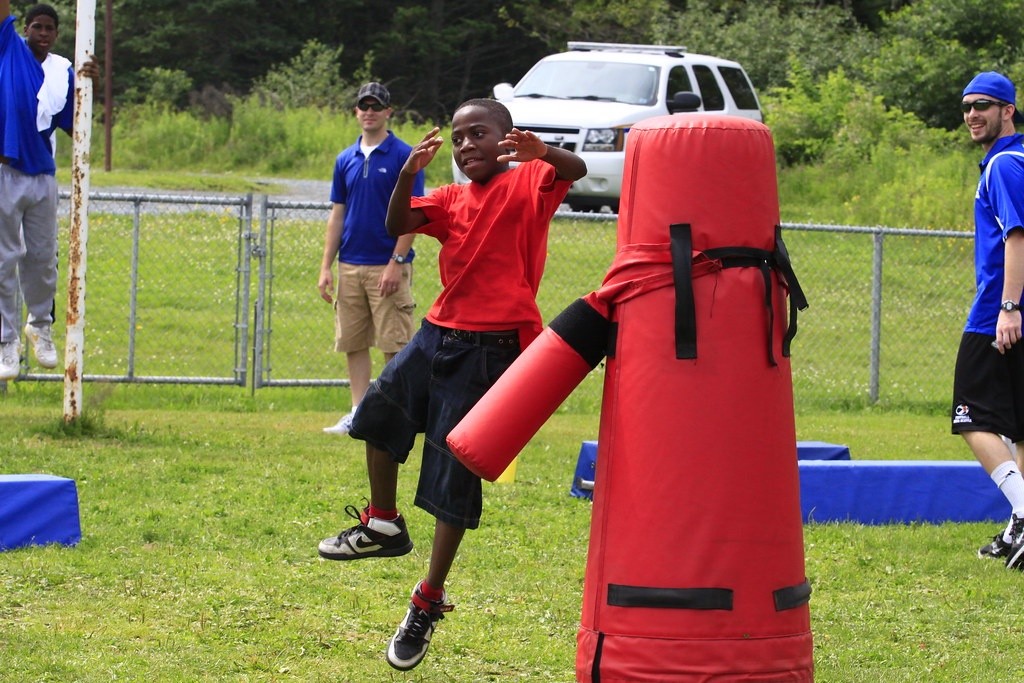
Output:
[0,0,99,379]
[952,70,1024,568]
[317,82,417,435]
[320,98,586,670]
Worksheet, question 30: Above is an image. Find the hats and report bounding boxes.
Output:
[356,82,391,108]
[961,71,1024,124]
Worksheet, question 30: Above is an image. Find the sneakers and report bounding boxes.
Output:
[0,336,23,381]
[323,410,356,435]
[978,528,1013,557]
[25,322,58,369]
[386,579,454,670]
[318,496,412,561]
[1006,513,1024,569]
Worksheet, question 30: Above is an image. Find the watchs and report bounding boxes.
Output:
[391,255,404,264]
[1001,301,1020,311]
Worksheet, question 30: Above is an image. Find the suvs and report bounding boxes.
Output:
[452,42,766,214]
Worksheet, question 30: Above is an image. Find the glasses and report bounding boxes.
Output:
[356,102,385,112]
[961,98,1006,112]
